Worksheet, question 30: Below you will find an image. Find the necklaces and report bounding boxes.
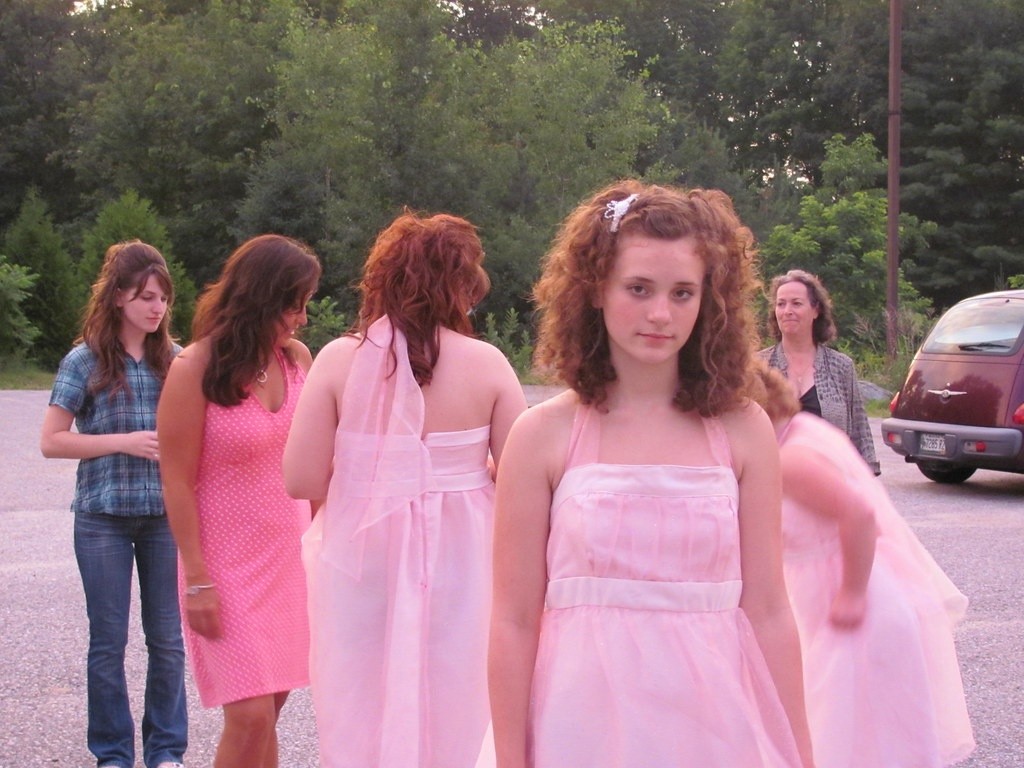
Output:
[784,353,813,383]
[256,369,268,383]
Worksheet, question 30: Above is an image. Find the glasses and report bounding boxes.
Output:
[462,287,476,316]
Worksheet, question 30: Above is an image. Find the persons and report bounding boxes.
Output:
[282,205,529,768]
[157,235,321,768]
[474,180,976,768]
[40,239,185,768]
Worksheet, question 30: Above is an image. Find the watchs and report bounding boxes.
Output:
[184,584,215,594]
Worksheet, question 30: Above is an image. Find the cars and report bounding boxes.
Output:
[880,289,1024,485]
